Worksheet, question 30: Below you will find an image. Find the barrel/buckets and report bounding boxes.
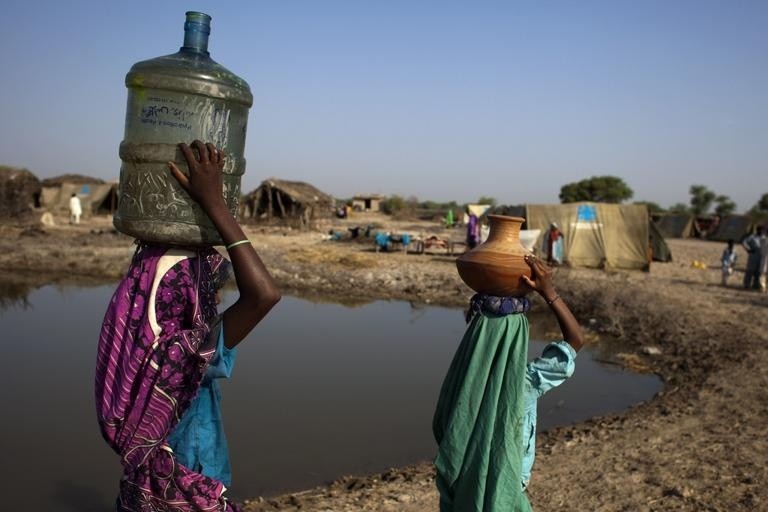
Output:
[114,11,253,245]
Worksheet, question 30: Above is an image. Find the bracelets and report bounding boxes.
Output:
[548,293,561,305]
[226,240,251,249]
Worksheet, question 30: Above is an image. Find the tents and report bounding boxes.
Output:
[502,202,675,269]
[649,210,768,245]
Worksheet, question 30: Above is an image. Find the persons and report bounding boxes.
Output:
[94,140,282,511]
[446,209,470,229]
[718,238,738,287]
[337,203,352,219]
[543,223,564,267]
[68,193,82,225]
[741,225,768,293]
[466,214,481,249]
[431,254,586,512]
